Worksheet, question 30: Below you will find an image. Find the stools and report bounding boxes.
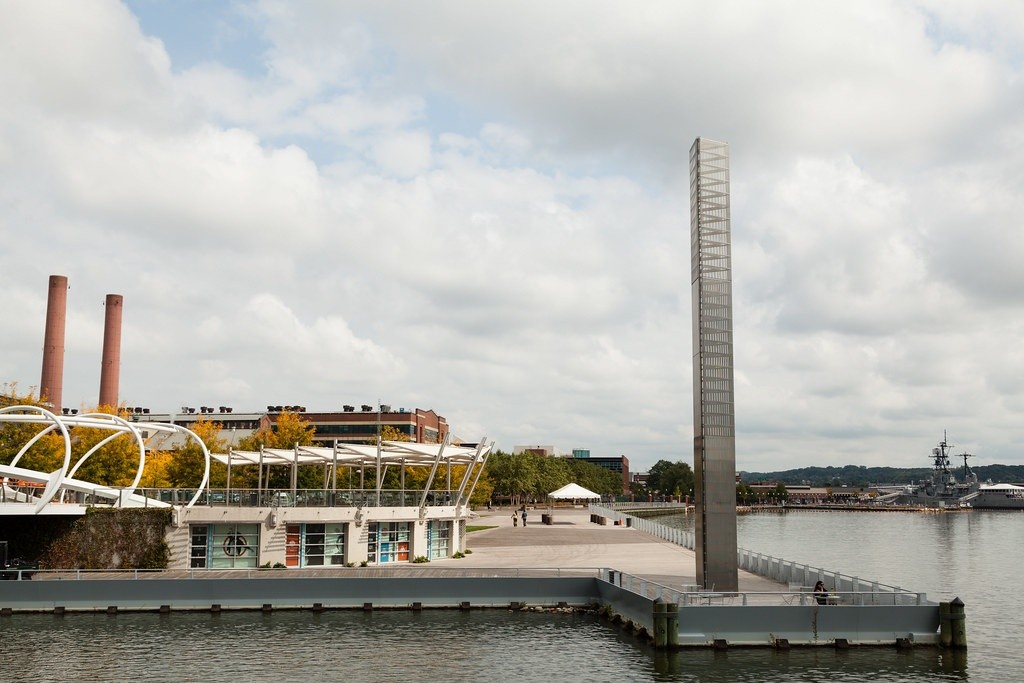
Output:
[590,514,607,525]
[542,514,553,524]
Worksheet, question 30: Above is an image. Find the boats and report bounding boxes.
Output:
[968,481,1024,510]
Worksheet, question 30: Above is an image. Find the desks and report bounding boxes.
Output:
[700,594,724,607]
[827,596,841,605]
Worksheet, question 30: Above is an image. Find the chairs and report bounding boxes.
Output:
[782,581,832,605]
[656,584,734,606]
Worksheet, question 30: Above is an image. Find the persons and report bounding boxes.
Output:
[487,501,492,509]
[813,581,837,605]
[522,510,527,526]
[0,559,23,580]
[511,510,518,526]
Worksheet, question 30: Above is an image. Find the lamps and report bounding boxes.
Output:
[356,510,364,526]
[270,509,278,527]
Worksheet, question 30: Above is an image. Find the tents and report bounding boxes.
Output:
[548,483,603,522]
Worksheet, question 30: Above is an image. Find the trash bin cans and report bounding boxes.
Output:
[626,518,632,527]
